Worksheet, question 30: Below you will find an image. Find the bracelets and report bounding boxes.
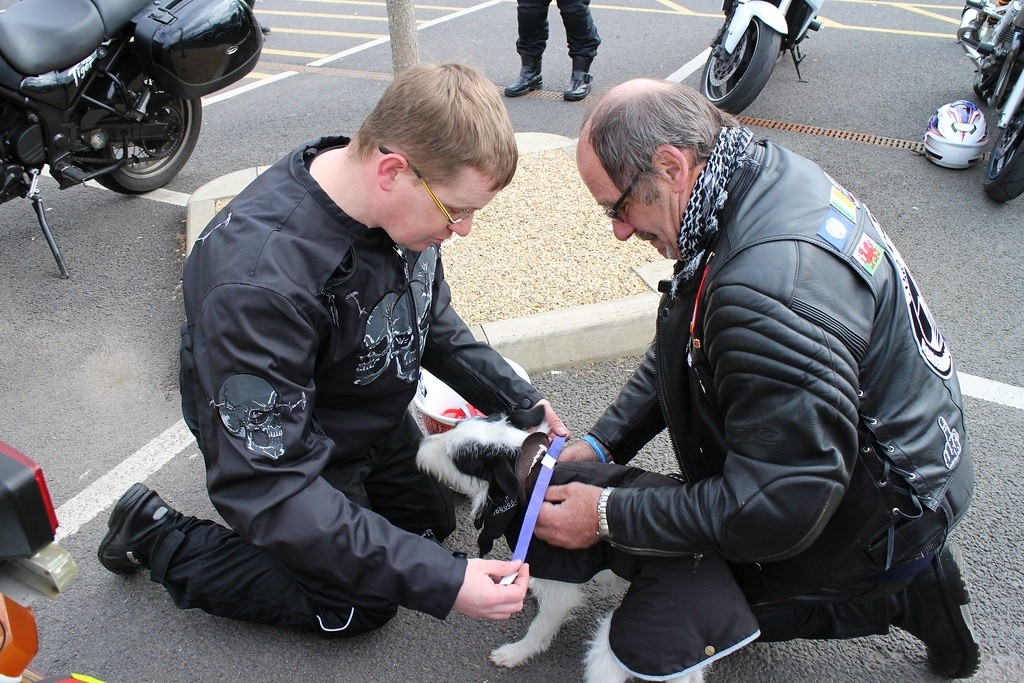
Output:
[597,485,616,542]
[581,435,609,463]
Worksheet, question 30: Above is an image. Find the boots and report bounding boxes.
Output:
[504,53,542,97]
[563,56,594,101]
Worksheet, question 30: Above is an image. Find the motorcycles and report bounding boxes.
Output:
[697,1,830,115]
[0,0,273,281]
[951,0,1024,206]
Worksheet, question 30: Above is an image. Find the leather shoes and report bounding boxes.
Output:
[899,542,982,680]
[97,482,183,577]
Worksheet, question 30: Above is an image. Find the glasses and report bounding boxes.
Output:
[378,144,474,225]
[602,143,689,223]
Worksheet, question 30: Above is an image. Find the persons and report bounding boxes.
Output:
[97,63,570,639]
[504,0,601,101]
[534,78,981,679]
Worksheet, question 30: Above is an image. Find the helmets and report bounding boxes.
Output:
[923,100,990,169]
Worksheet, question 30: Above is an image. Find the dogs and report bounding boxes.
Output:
[415,404,708,683]
